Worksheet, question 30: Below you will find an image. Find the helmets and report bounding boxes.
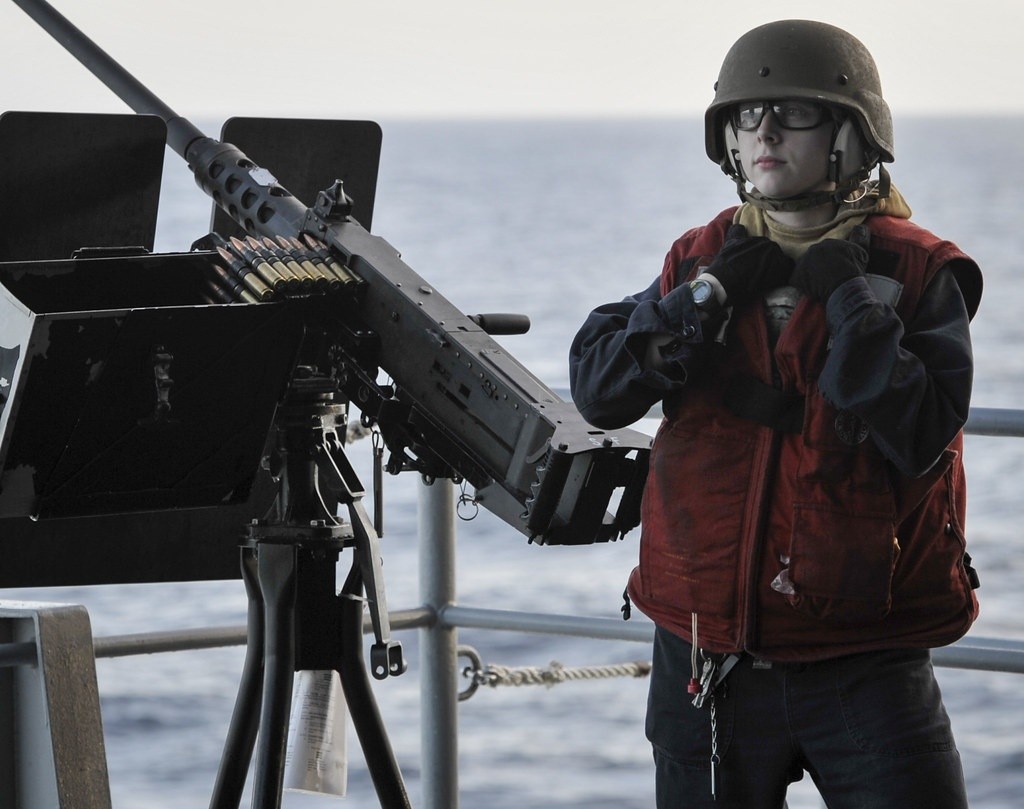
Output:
[704,20,896,165]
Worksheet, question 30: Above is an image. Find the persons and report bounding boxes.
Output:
[570,19,985,809]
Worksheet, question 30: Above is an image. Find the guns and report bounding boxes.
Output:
[14,0,653,549]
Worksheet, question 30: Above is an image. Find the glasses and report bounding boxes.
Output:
[727,99,839,130]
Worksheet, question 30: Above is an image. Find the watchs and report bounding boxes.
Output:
[690,279,723,318]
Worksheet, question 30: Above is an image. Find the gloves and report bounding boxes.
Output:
[702,221,792,307]
[789,237,873,307]
